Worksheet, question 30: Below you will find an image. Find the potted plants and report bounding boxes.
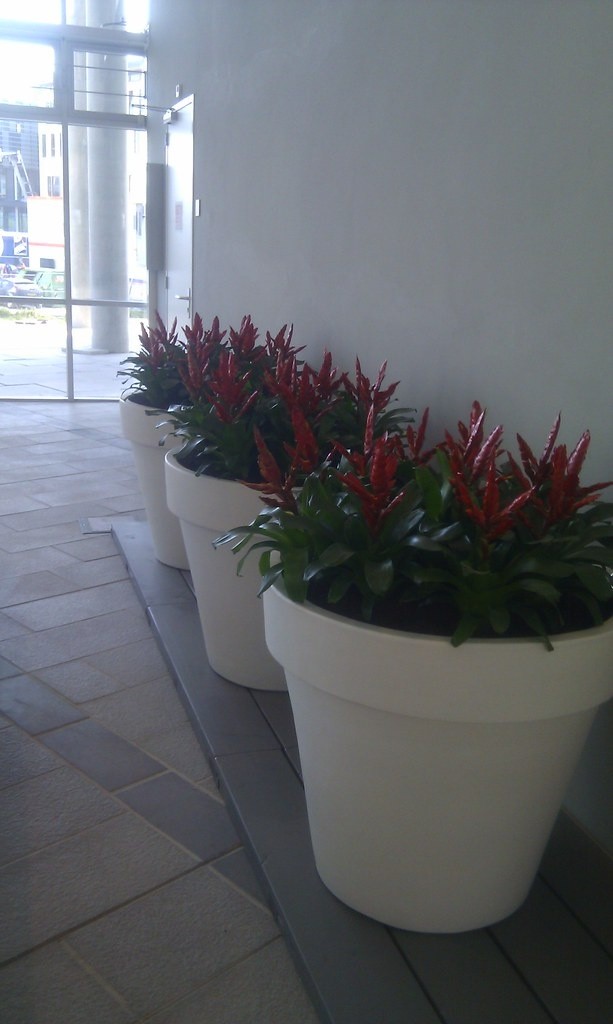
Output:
[218,390,612,936]
[118,305,202,571]
[143,314,396,692]
